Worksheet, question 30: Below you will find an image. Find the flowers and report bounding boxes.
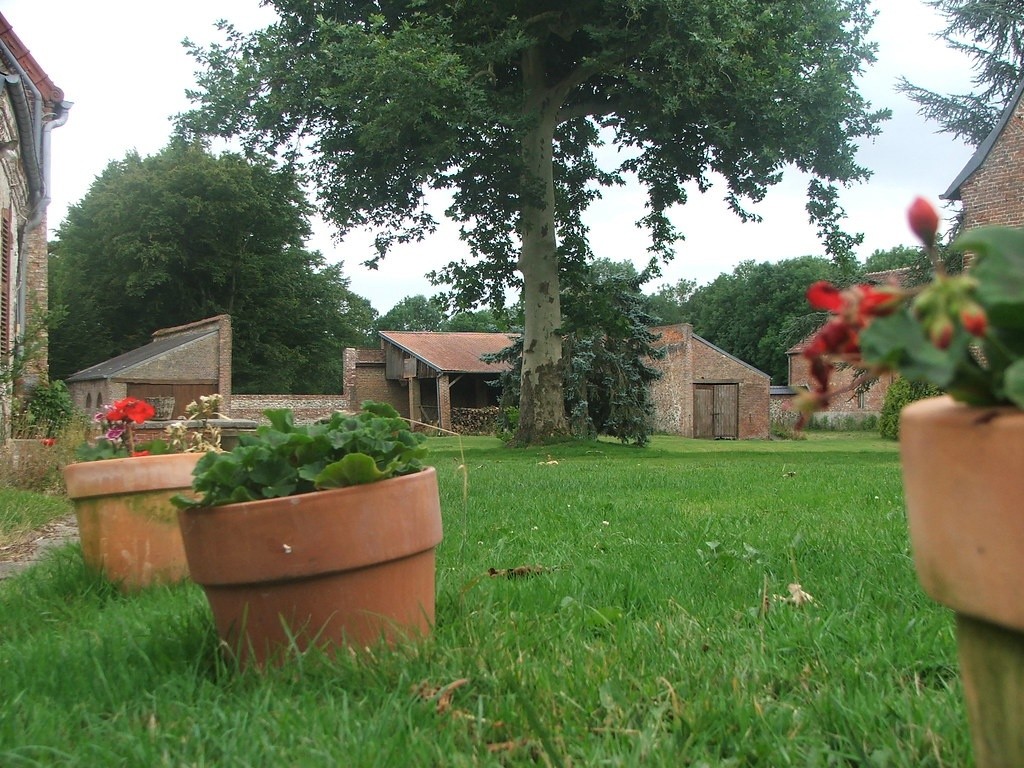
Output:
[73,393,232,461]
[778,194,1024,428]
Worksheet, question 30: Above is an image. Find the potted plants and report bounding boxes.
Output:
[169,399,444,672]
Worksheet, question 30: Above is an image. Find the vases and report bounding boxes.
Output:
[62,452,210,593]
[898,394,1024,768]
